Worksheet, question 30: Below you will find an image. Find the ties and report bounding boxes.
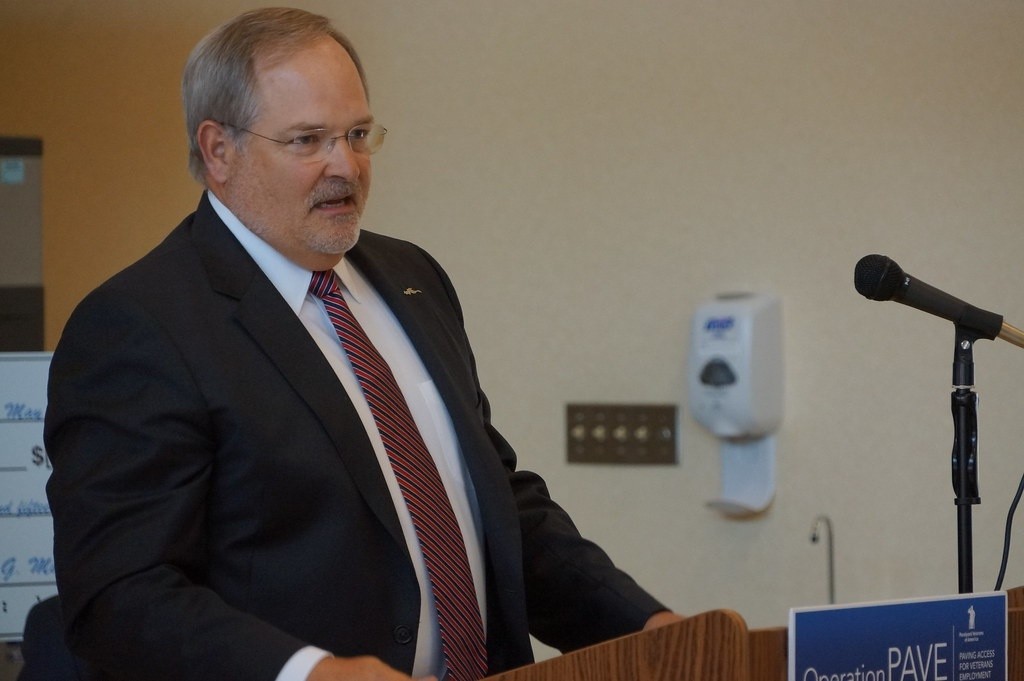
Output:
[308,267,488,681]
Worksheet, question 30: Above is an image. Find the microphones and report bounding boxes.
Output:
[855,254,1024,350]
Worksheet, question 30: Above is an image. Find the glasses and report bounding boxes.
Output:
[216,119,388,160]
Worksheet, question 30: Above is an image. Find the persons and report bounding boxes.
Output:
[44,6,689,680]
